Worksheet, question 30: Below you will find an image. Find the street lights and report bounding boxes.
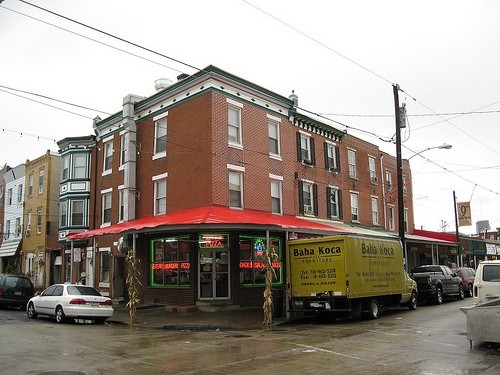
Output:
[396,143,452,268]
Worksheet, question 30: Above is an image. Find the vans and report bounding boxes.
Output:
[473,260,500,304]
[450,268,476,297]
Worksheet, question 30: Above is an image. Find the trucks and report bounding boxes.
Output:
[288,234,418,321]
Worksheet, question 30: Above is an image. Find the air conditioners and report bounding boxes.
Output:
[24,230,30,236]
[372,177,378,183]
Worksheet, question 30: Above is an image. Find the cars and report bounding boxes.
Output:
[27,283,113,324]
[157,270,220,284]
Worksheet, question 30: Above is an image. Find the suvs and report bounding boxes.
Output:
[0,272,34,312]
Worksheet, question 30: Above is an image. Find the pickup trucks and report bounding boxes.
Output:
[409,265,464,304]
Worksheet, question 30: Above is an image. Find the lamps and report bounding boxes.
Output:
[19,249,27,255]
